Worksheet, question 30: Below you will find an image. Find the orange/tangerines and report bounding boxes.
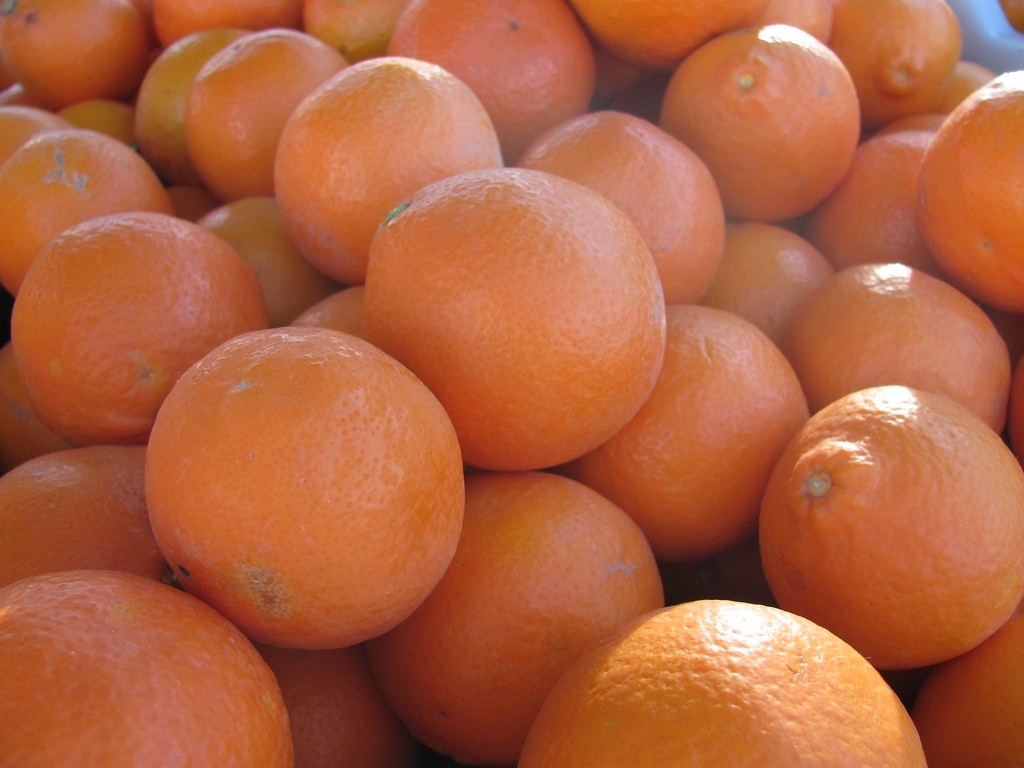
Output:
[0,1,1024,768]
